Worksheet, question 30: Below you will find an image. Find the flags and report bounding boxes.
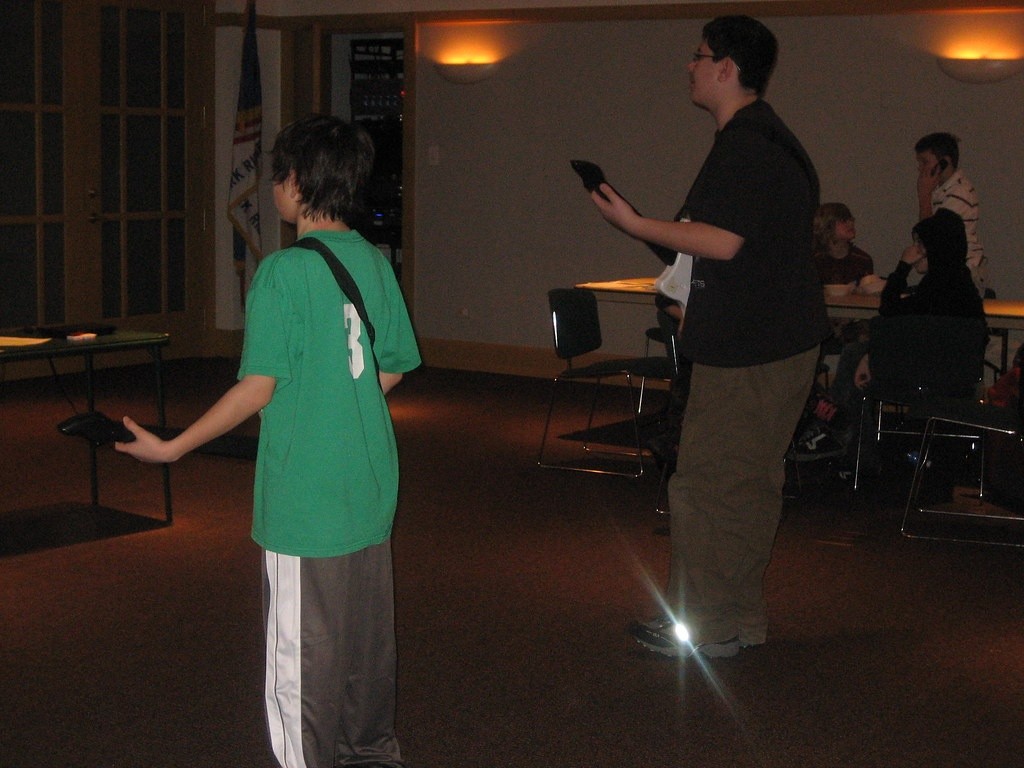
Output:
[226,0,262,318]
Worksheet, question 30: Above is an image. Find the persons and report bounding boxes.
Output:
[914,132,986,302]
[812,203,880,296]
[784,207,988,493]
[655,204,693,453]
[591,14,834,657]
[114,114,423,768]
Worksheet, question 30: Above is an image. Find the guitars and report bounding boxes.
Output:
[55,411,259,461]
[569,159,694,320]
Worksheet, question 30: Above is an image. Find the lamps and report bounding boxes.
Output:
[434,62,500,84]
[937,57,1024,83]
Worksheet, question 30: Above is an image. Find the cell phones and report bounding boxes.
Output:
[931,159,947,176]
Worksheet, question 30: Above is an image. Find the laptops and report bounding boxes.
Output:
[35,322,117,337]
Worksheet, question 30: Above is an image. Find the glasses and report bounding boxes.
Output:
[692,51,741,71]
[835,216,857,224]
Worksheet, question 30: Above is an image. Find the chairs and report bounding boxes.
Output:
[843,288,1024,552]
[536,288,828,514]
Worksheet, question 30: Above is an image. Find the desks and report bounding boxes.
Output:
[575,277,1024,429]
[0,326,173,523]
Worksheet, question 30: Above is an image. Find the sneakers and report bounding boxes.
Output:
[793,425,850,462]
[812,392,852,434]
[628,614,747,659]
[832,456,887,488]
